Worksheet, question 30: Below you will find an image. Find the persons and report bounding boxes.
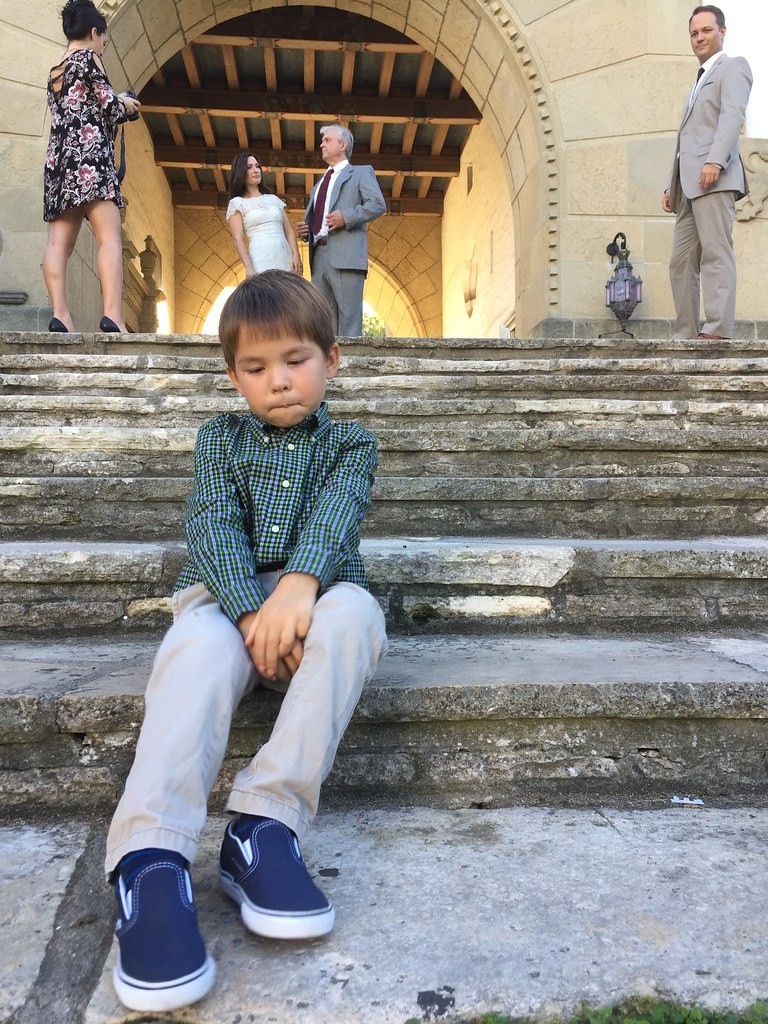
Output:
[225,151,304,278]
[294,124,386,338]
[41,0,143,334]
[99,265,391,1011]
[657,2,754,341]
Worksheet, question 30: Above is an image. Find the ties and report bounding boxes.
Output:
[311,169,334,236]
[692,68,705,99]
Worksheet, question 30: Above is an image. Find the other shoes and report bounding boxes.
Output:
[697,333,730,339]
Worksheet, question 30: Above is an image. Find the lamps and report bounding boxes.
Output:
[600,232,642,338]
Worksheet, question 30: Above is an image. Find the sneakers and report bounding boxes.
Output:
[216,817,334,939]
[112,860,216,1013]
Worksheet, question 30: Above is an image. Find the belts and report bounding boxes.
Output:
[317,238,327,246]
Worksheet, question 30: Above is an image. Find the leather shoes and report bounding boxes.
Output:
[49,317,69,332]
[99,316,120,332]
